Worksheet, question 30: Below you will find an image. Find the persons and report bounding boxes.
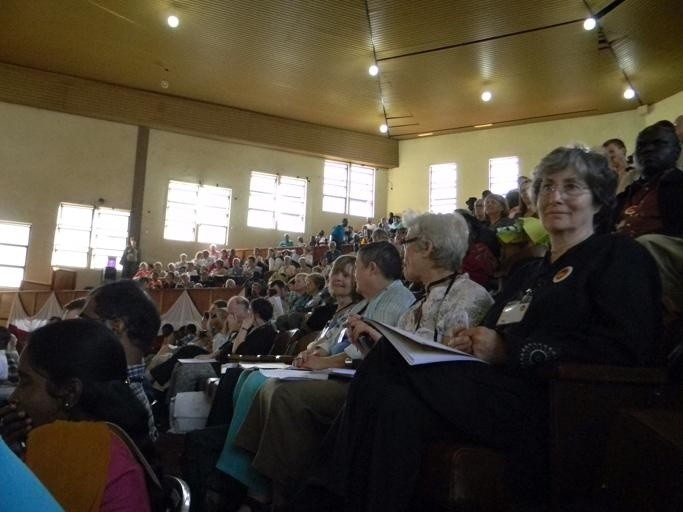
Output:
[0,118,683,511]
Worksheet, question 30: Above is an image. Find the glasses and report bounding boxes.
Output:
[540,180,590,196]
[399,237,417,248]
